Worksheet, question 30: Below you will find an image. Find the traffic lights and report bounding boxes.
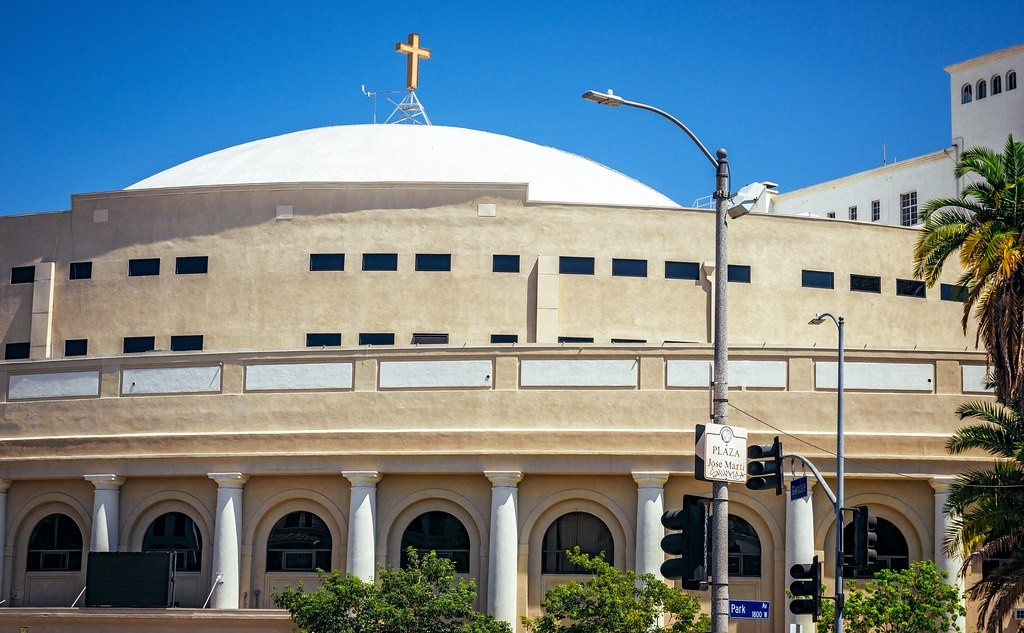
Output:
[788,557,822,623]
[660,493,706,590]
[748,436,784,496]
[854,506,878,569]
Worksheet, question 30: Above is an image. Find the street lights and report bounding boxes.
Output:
[808,312,846,633]
[580,88,767,633]
